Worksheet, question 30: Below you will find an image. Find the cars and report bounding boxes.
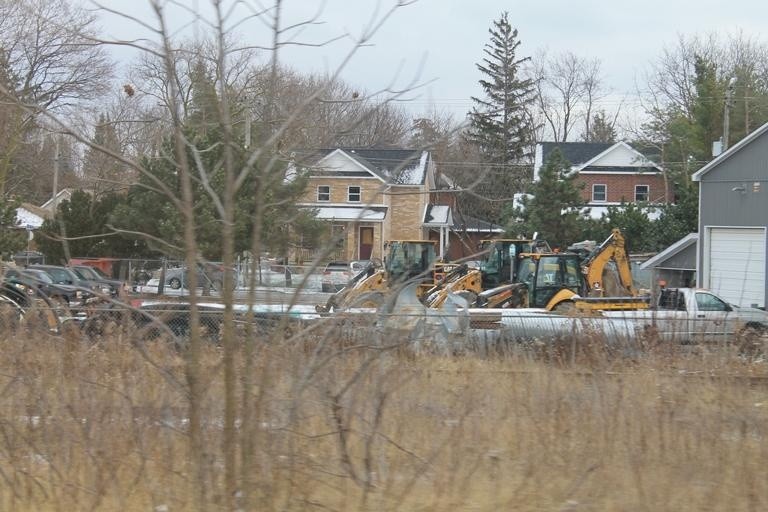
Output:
[165,261,243,290]
[0,262,130,314]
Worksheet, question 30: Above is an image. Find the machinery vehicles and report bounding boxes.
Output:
[314,227,656,317]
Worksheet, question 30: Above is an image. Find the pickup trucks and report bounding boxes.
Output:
[591,287,768,348]
